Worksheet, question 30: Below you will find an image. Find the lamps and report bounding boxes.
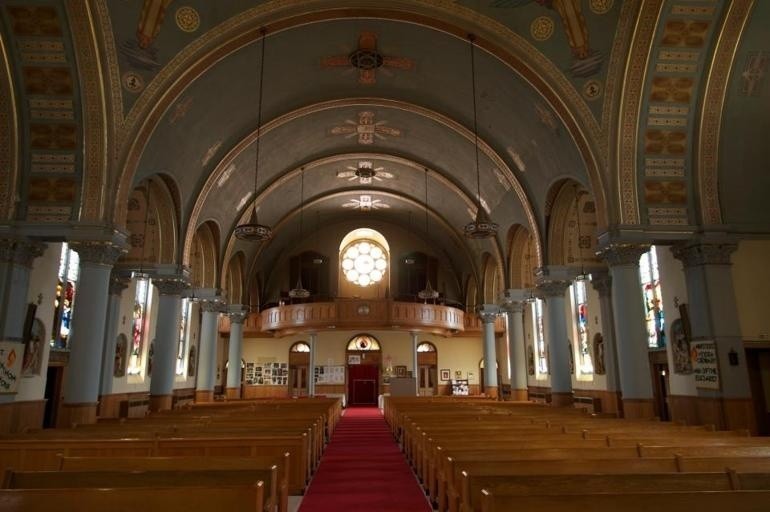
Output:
[232,26,311,299]
[417,35,500,300]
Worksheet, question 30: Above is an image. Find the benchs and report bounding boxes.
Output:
[0,393,342,512]
[382,394,770,512]
